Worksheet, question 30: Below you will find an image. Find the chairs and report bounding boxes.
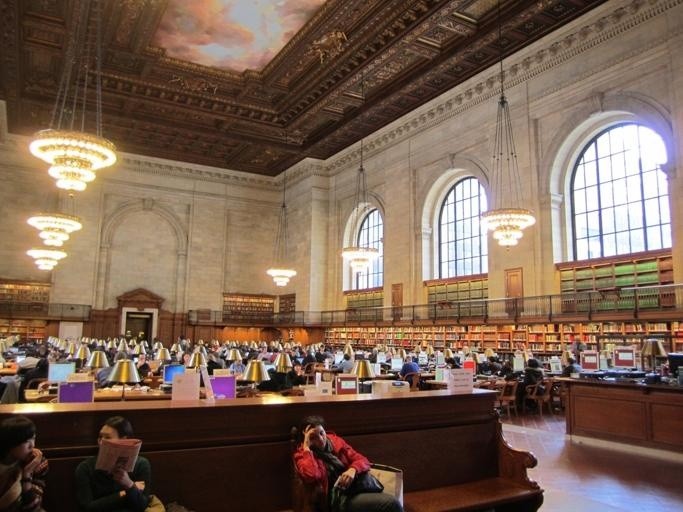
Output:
[304,361,566,421]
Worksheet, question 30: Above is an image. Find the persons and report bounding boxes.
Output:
[291,415,403,510]
[0,331,585,417]
[74,416,151,512]
[0,417,49,511]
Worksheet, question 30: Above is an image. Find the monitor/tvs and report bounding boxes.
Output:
[296,351,563,381]
[163,364,186,384]
[17,356,26,364]
[48,362,76,386]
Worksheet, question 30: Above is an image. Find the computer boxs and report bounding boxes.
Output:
[48,386,58,395]
[159,384,172,393]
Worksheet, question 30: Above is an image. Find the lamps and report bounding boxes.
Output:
[27,178,84,250]
[478,0,537,247]
[27,0,118,195]
[339,73,379,273]
[24,245,68,271]
[262,127,298,287]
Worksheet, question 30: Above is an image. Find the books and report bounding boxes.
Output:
[0,319,45,344]
[0,283,49,303]
[223,297,294,322]
[94,437,142,474]
[321,323,682,370]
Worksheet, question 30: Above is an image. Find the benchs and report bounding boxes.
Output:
[0,396,543,512]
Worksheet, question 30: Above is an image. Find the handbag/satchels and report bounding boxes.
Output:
[355,473,384,493]
[366,463,404,509]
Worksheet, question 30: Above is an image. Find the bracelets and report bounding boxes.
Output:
[20,477,32,482]
